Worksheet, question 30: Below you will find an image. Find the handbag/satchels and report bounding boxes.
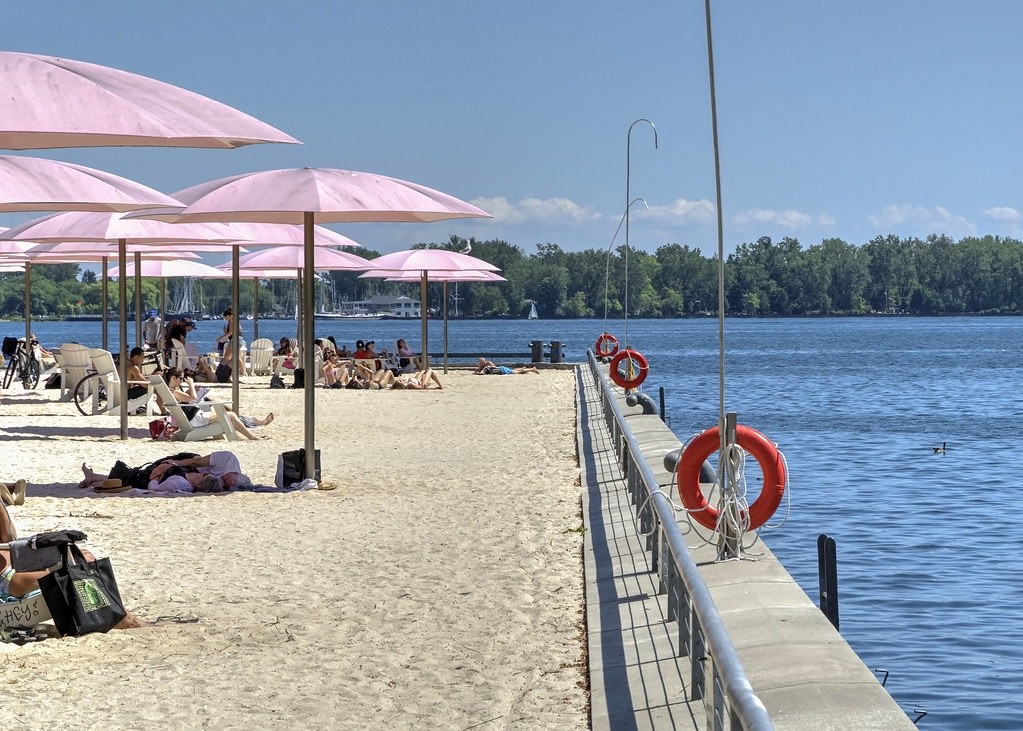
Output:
[275,448,321,489]
[270,374,285,389]
[37,542,127,637]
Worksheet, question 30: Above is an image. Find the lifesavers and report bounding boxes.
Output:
[596,333,620,358]
[676,423,786,531]
[610,349,650,390]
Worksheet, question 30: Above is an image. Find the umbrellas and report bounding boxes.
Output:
[0,212,258,440]
[120,167,494,480]
[0,155,380,364]
[0,51,302,149]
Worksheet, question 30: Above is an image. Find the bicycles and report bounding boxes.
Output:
[74,369,107,416]
[2,340,41,390]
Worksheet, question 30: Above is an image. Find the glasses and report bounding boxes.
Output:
[202,473,214,480]
[177,376,183,381]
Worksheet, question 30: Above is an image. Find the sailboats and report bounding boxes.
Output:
[527,302,539,320]
[164,276,387,320]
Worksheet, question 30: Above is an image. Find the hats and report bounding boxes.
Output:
[149,309,156,318]
[186,322,197,330]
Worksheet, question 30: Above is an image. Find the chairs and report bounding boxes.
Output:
[18,338,432,442]
[0,540,86,630]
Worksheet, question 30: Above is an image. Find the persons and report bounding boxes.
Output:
[356,250,508,374]
[116,305,274,440]
[274,336,443,389]
[82,450,254,494]
[0,498,134,630]
[475,357,539,375]
[30,333,53,358]
